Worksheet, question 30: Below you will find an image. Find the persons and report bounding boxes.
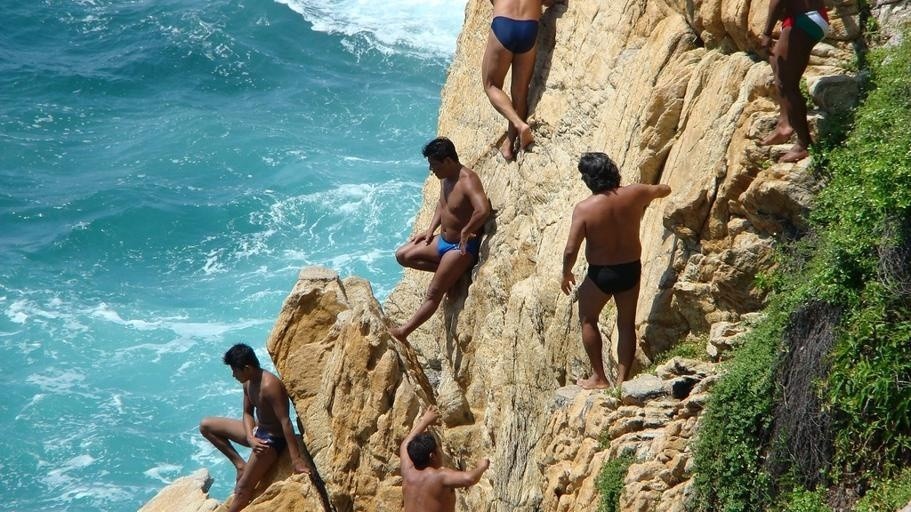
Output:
[480,0,560,163]
[560,149,674,392]
[396,403,493,512]
[198,342,318,511]
[757,1,833,166]
[385,134,494,342]
